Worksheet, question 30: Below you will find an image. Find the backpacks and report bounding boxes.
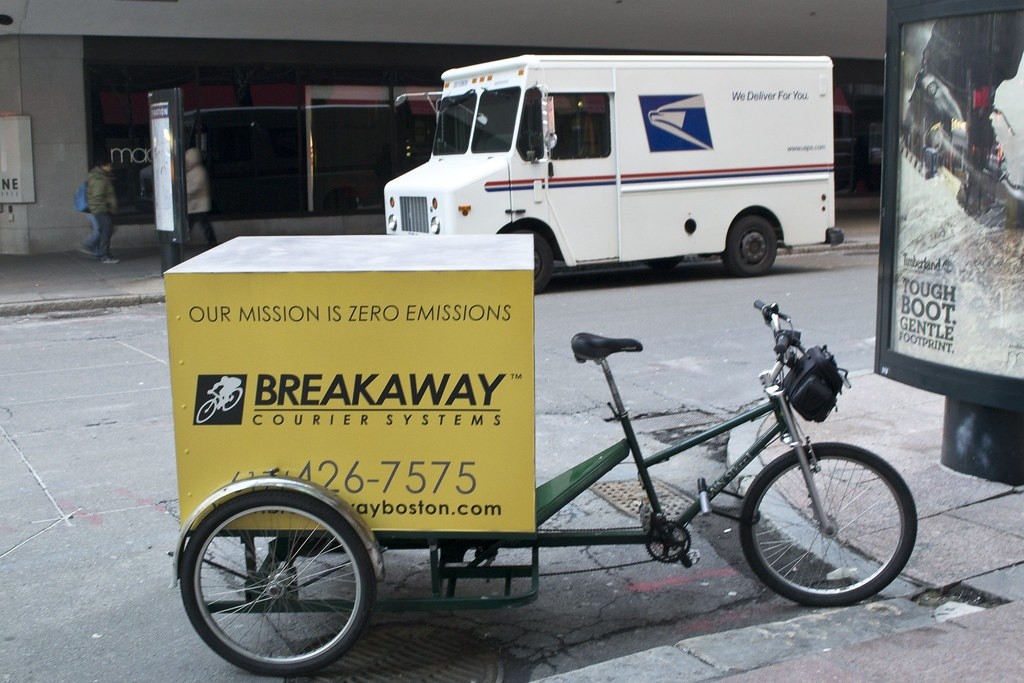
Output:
[73,181,91,213]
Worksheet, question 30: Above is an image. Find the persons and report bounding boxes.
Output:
[185,148,217,247]
[79,157,120,264]
[139,165,154,221]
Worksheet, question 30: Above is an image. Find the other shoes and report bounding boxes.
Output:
[78,243,99,256]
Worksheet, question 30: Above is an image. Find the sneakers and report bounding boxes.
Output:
[101,257,119,264]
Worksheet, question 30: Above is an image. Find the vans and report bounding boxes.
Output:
[380,51,844,297]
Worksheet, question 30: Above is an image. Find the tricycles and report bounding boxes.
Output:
[156,232,919,678]
